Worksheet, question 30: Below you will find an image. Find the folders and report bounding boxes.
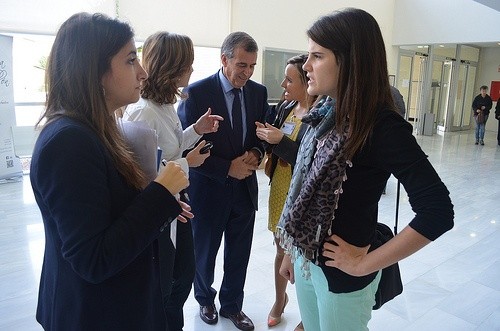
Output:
[157,148,162,172]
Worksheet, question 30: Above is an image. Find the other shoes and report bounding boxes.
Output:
[480,140,485,145]
[475,138,479,144]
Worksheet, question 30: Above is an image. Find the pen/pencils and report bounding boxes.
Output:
[161,159,190,201]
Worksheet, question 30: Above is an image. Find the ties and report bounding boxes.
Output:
[230,88,243,154]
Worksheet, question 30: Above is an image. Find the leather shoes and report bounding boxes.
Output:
[220,306,254,331]
[199,301,218,323]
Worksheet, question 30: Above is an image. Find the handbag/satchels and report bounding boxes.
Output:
[370,223,403,310]
[477,111,484,123]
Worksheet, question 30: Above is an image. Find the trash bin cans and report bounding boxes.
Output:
[423,113,435,135]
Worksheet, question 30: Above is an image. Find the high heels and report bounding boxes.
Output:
[294,322,304,331]
[267,293,289,326]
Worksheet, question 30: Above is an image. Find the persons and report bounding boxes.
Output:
[471,85,493,146]
[30,13,198,331]
[282,7,455,331]
[114,30,224,314]
[254,52,327,331]
[381,83,407,195]
[494,97,500,146]
[176,31,272,331]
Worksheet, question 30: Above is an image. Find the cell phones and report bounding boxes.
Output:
[200,140,214,154]
